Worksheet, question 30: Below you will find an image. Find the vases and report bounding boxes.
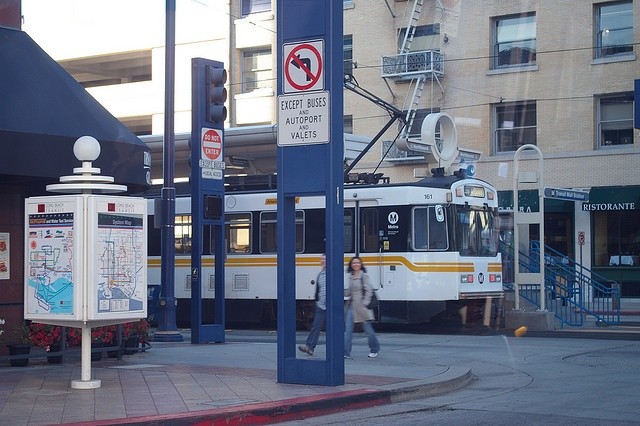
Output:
[107,338,127,358]
[47,345,63,363]
[7,345,30,367]
[91,344,102,361]
[125,334,139,354]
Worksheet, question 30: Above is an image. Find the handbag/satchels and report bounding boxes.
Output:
[367,292,378,309]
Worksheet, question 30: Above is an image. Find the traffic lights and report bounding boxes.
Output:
[206,65,227,124]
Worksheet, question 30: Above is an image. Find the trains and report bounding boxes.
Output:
[144,164,503,331]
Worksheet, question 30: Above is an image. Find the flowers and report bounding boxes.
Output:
[65,328,81,349]
[112,323,133,337]
[14,323,47,352]
[133,320,148,342]
[47,325,63,351]
[92,327,112,343]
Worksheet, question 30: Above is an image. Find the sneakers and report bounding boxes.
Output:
[299,345,314,355]
[368,350,381,357]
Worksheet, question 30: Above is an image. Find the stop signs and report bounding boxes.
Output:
[199,126,223,161]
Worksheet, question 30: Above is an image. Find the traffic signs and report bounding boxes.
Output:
[276,90,329,145]
[543,187,589,202]
[197,159,225,180]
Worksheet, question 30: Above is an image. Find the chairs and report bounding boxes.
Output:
[601,253,607,266]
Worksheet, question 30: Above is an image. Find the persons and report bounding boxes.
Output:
[297,252,327,356]
[343,256,381,359]
